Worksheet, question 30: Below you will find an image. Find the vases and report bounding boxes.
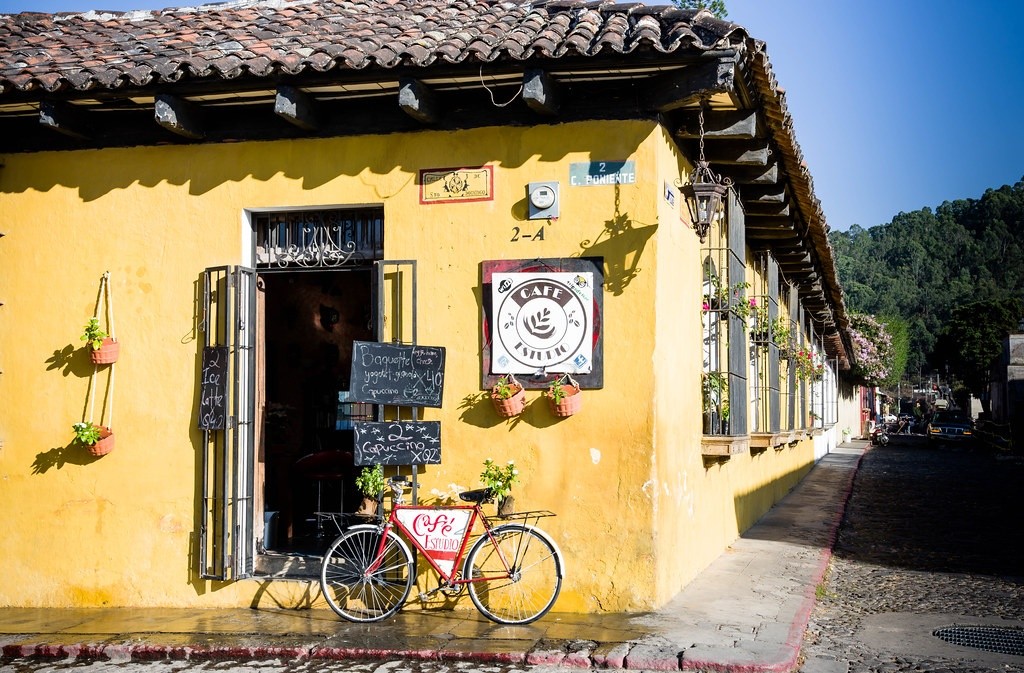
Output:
[779,349,788,359]
[702,412,715,435]
[547,385,582,418]
[719,420,730,435]
[358,495,378,515]
[87,341,119,364]
[81,425,114,458]
[722,300,730,311]
[844,435,852,443]
[708,298,719,310]
[751,329,767,346]
[497,494,514,517]
[813,374,823,380]
[764,332,777,350]
[490,384,525,418]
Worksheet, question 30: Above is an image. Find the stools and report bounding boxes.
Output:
[295,448,350,551]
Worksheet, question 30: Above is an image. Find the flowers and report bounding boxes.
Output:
[356,467,385,495]
[480,459,518,501]
[71,421,100,445]
[545,374,567,405]
[496,375,519,398]
[80,317,105,346]
[701,272,824,420]
[843,427,853,435]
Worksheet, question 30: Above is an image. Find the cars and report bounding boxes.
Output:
[897,413,909,423]
[926,409,975,450]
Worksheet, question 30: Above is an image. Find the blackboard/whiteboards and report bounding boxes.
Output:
[353,419,444,467]
[351,338,448,407]
[197,343,230,432]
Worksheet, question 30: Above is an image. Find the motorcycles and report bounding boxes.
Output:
[870,423,889,447]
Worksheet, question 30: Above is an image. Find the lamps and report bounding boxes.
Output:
[679,104,726,244]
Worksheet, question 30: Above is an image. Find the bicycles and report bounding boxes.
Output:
[320,475,564,626]
